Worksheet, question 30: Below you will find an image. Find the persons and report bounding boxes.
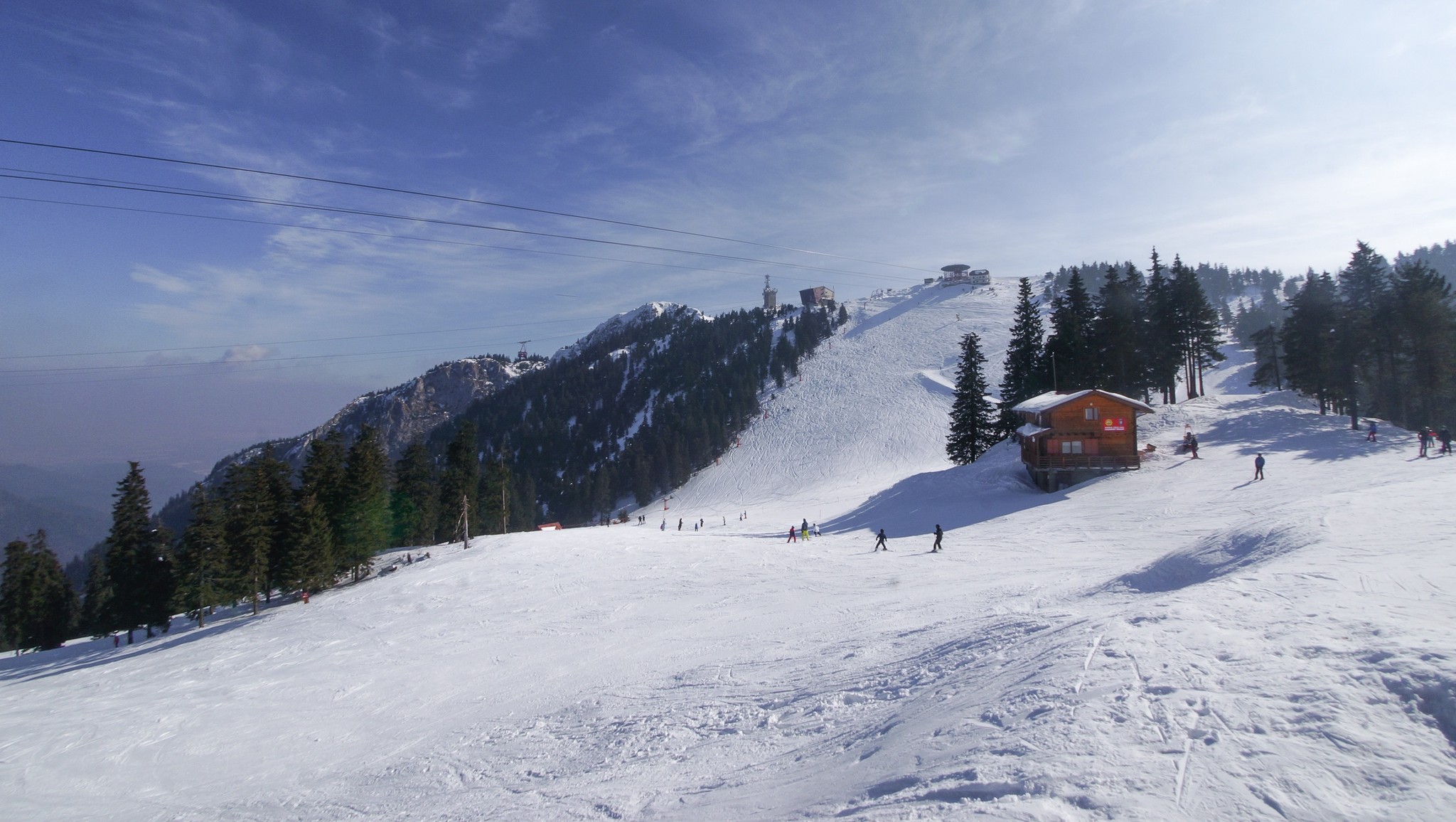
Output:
[874,528,887,552]
[1255,453,1265,480]
[678,518,683,530]
[112,636,120,648]
[788,526,796,542]
[1191,438,1199,459]
[723,516,725,525]
[1365,421,1377,442]
[695,523,698,531]
[1428,429,1434,447]
[407,552,411,564]
[638,514,645,525]
[812,524,821,536]
[801,519,809,541]
[606,516,609,527]
[931,524,943,552]
[1417,426,1430,457]
[1437,425,1452,457]
[700,519,703,527]
[740,510,747,520]
[662,518,665,530]
